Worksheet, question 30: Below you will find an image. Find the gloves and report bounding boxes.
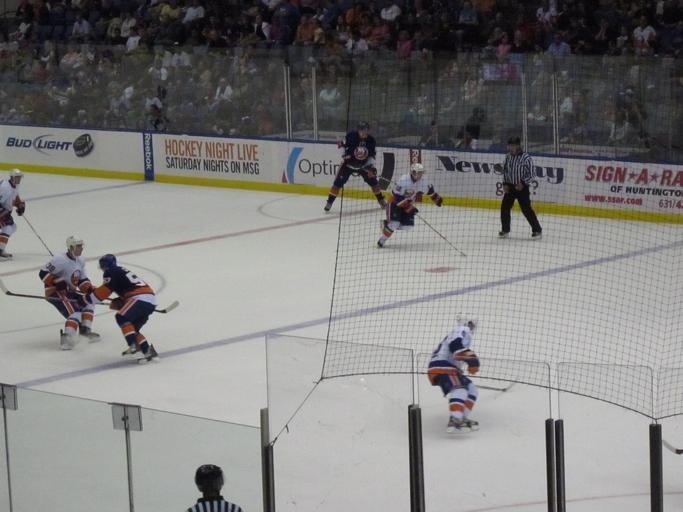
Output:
[453,347,480,375]
[367,164,377,180]
[430,192,443,208]
[74,290,103,310]
[53,276,70,295]
[109,296,127,310]
[396,198,419,217]
[14,200,26,216]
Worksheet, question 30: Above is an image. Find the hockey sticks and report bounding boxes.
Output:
[108,297,179,313]
[1,281,110,305]
[475,381,518,392]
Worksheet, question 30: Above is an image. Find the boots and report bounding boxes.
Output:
[0,247,14,258]
[375,192,388,211]
[324,193,337,212]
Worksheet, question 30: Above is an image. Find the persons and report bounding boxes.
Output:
[37,233,101,351]
[375,161,443,248]
[183,464,244,512]
[0,167,26,262]
[76,253,160,364]
[496,135,542,241]
[324,120,388,213]
[426,312,481,429]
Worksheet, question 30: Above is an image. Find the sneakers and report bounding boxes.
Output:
[121,341,142,356]
[498,230,511,236]
[78,325,100,339]
[59,330,78,346]
[447,415,479,429]
[531,228,543,237]
[137,343,158,362]
[377,237,385,249]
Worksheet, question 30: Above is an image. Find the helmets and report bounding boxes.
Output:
[66,233,84,251]
[507,133,522,147]
[455,310,480,328]
[99,254,117,270]
[195,463,224,492]
[357,120,370,131]
[8,168,23,178]
[410,162,425,173]
